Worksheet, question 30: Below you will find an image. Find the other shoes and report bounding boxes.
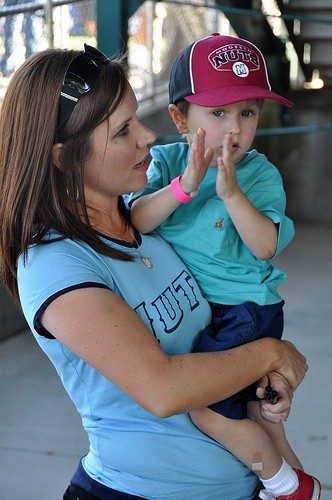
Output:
[259,468,321,500]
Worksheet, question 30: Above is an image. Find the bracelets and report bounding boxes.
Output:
[171,175,200,203]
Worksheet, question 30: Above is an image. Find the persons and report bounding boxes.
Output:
[0,49,308,500]
[122,32,321,500]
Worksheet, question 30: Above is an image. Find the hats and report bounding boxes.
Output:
[168,32,294,108]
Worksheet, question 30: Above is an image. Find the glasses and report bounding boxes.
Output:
[54,43,109,143]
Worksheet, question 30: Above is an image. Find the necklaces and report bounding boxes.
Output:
[79,200,153,268]
